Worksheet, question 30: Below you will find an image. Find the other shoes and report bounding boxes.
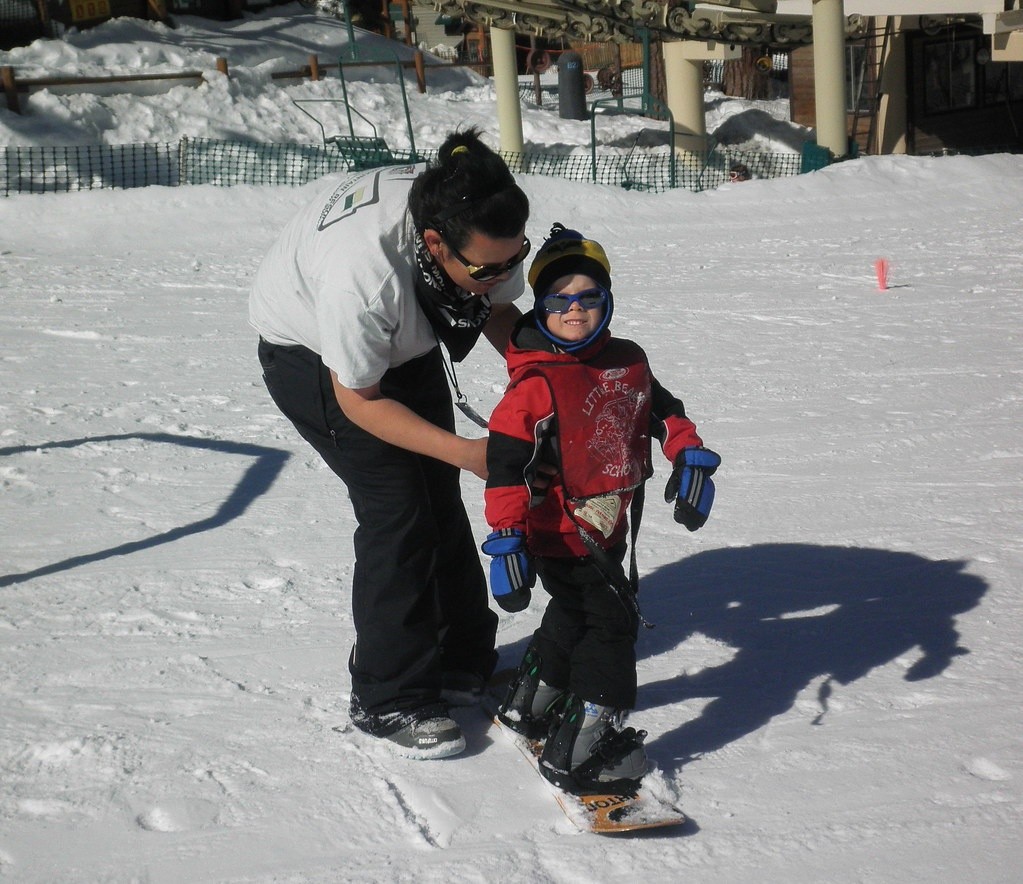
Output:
[542,700,648,782]
[502,665,572,722]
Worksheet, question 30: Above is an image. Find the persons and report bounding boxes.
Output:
[249,130,531,759]
[480,230,720,793]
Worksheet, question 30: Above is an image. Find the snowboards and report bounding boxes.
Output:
[480,665,688,834]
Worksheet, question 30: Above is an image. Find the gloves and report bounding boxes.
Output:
[664,446,721,531]
[481,528,536,613]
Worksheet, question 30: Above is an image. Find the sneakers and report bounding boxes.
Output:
[357,716,465,761]
[440,664,484,706]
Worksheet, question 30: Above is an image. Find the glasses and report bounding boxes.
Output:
[541,288,607,314]
[437,228,531,282]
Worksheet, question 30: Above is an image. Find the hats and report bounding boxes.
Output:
[528,222,612,290]
